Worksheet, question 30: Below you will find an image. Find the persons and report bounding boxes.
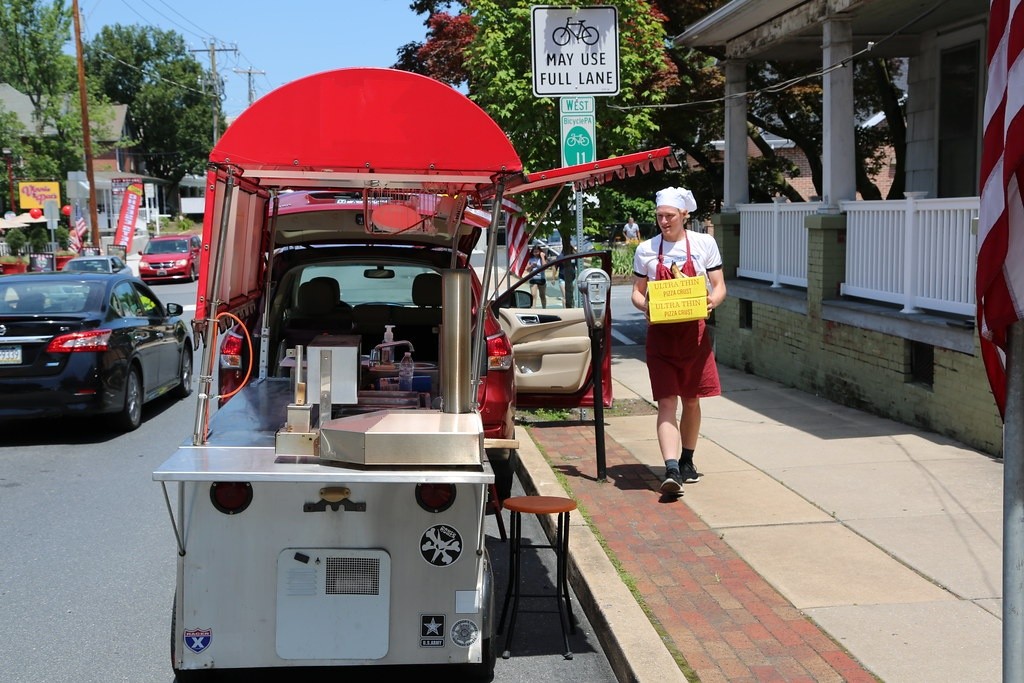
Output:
[526,246,548,309]
[622,218,640,243]
[551,246,578,308]
[632,187,726,495]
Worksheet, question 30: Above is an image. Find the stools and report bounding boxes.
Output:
[498,496,577,658]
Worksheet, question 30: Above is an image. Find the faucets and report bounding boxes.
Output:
[374,339,416,362]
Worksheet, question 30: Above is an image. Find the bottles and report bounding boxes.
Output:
[398,352,414,391]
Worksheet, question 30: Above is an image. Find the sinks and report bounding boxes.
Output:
[375,363,437,370]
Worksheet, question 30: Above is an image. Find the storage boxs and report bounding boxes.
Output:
[648,297,707,324]
[647,276,706,301]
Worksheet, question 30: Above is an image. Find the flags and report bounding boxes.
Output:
[68,206,88,254]
[976,0,1024,422]
[502,196,533,278]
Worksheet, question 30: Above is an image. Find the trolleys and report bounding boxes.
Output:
[153,67,526,682]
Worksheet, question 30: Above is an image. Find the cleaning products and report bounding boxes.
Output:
[380,324,397,364]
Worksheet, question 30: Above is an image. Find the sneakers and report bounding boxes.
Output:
[678,459,700,482]
[660,468,680,496]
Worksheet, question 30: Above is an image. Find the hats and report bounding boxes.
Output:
[656,187,697,213]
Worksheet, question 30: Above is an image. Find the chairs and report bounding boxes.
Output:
[307,276,353,344]
[16,292,45,313]
[391,273,442,359]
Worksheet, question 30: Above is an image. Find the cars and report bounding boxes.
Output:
[216,189,612,498]
[0,272,194,433]
[49,255,132,310]
[138,233,201,285]
[594,208,660,242]
[486,210,532,245]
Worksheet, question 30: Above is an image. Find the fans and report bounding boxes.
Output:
[434,193,468,240]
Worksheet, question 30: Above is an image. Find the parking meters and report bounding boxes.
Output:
[577,267,610,483]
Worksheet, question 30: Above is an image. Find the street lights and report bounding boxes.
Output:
[2,148,15,213]
